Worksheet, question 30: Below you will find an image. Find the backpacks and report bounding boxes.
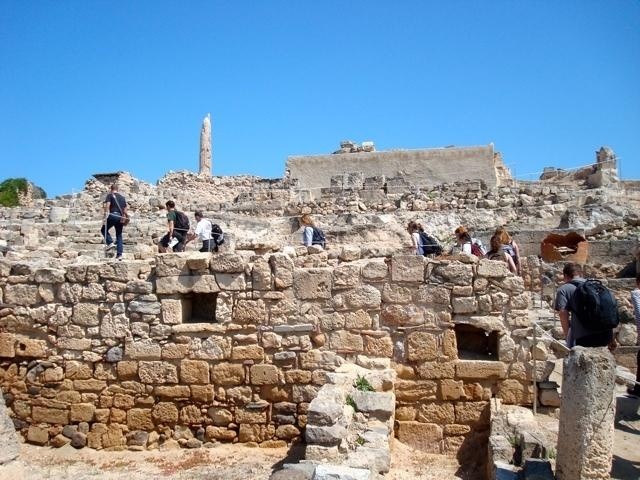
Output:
[568,279,620,331]
[471,243,483,256]
[419,231,442,255]
[502,243,517,261]
[174,210,190,232]
[211,223,224,246]
[312,228,325,245]
[488,252,506,261]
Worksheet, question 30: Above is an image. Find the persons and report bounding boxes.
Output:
[495,225,522,275]
[300,213,325,246]
[158,200,190,252]
[627,275,640,397]
[186,212,225,252]
[483,236,516,273]
[405,220,443,256]
[455,226,487,252]
[458,233,483,257]
[554,263,620,419]
[101,184,128,261]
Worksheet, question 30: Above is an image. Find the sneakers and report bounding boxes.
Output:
[105,242,115,251]
[627,383,639,399]
[116,255,122,260]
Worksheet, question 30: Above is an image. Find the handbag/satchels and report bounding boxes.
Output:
[119,211,130,225]
